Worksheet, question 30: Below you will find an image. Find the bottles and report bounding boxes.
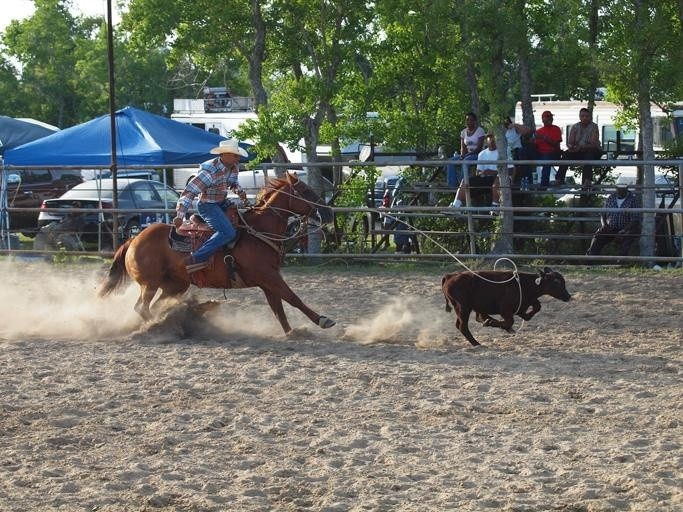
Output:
[520,178,525,192]
[438,145,445,160]
[525,176,529,192]
[454,150,459,157]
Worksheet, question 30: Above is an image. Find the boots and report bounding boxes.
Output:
[184,256,193,265]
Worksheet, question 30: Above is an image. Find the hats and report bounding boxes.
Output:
[210,140,248,157]
[616,176,628,188]
[542,111,554,117]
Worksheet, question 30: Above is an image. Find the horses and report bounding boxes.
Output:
[97,170,336,340]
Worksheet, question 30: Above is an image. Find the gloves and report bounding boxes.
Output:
[238,190,246,200]
[173,211,185,228]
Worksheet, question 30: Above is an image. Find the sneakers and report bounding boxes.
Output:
[480,227,489,237]
[556,179,566,186]
[490,205,500,215]
[380,243,390,251]
[441,202,464,215]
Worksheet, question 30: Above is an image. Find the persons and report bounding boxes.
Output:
[173,140,251,270]
[379,189,392,253]
[442,108,600,215]
[585,177,641,255]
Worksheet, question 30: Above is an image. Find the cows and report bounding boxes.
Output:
[442,266,570,347]
